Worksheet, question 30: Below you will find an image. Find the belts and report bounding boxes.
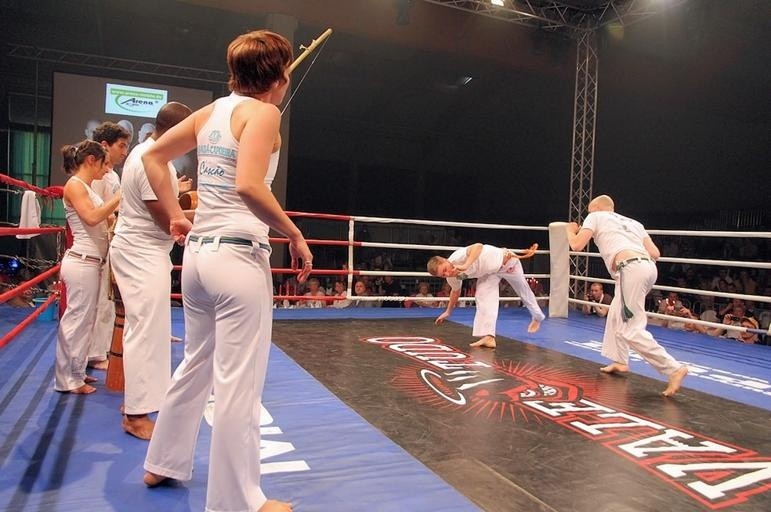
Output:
[617,257,650,267]
[187,236,271,254]
[67,252,100,264]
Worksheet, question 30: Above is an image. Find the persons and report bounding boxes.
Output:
[340,248,393,273]
[110,101,194,440]
[83,122,131,369]
[734,305,743,318]
[378,275,402,307]
[53,139,121,394]
[583,282,613,317]
[711,268,734,290]
[677,268,712,301]
[716,298,760,326]
[412,282,438,308]
[727,283,737,293]
[296,278,327,309]
[678,306,725,336]
[658,291,684,318]
[328,281,353,309]
[566,195,688,396]
[143,31,313,512]
[719,279,728,293]
[426,243,545,349]
[734,270,759,295]
[436,282,452,298]
[712,314,763,345]
[344,281,374,309]
[8,281,35,309]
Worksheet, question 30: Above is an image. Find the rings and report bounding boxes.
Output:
[305,261,313,266]
